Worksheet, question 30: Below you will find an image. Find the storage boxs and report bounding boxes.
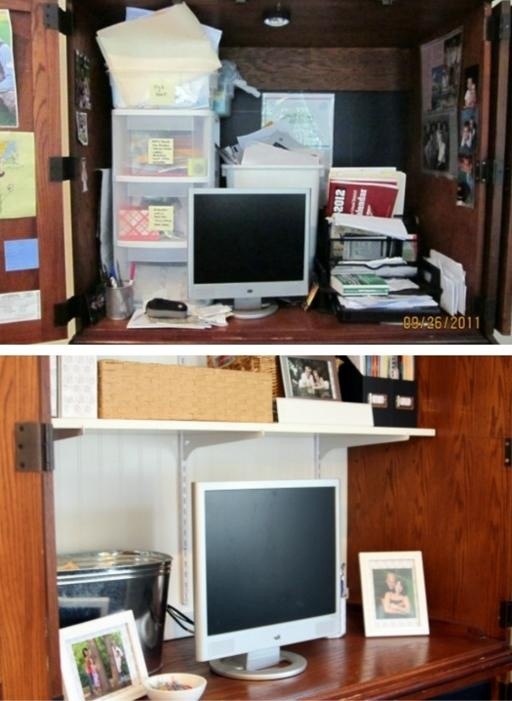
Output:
[106,61,221,307]
[220,161,328,279]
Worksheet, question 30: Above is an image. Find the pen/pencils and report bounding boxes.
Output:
[101,262,140,286]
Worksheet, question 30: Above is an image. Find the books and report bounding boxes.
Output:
[94,2,223,108]
[126,305,237,328]
[325,167,469,319]
[347,355,415,407]
[217,125,320,166]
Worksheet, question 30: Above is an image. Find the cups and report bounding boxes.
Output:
[106,280,134,321]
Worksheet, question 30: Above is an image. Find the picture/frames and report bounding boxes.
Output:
[358,550,433,638]
[57,610,151,701]
[278,355,344,402]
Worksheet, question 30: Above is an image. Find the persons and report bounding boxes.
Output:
[296,364,331,398]
[82,642,125,698]
[376,572,412,618]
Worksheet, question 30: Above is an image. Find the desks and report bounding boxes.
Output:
[54,603,512,701]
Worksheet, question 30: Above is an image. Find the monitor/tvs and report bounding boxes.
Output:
[189,187,311,319]
[193,479,349,681]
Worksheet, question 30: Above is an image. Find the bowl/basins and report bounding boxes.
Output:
[145,672,207,701]
